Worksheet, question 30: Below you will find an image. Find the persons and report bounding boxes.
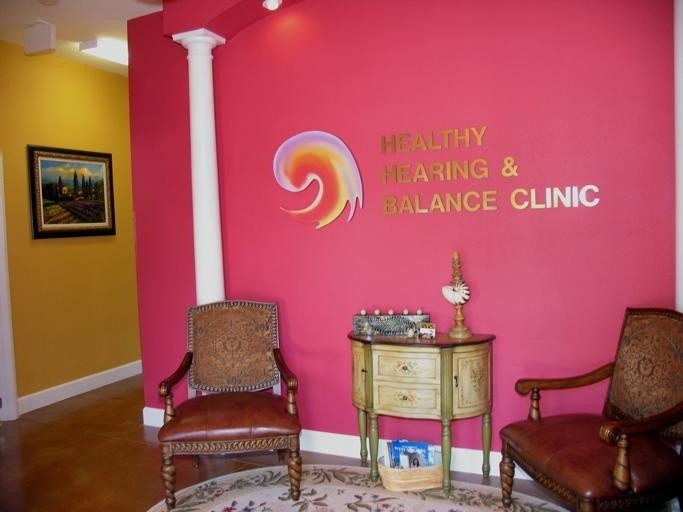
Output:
[411,458,419,468]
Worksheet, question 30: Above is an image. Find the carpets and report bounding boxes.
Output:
[144,463,573,512]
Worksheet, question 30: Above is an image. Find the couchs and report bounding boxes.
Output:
[157,299,302,512]
[498,305,683,512]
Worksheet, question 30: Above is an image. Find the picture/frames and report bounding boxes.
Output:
[26,142,118,241]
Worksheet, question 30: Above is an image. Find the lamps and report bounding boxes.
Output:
[262,0,283,12]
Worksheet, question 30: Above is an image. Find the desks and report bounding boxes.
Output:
[346,330,497,498]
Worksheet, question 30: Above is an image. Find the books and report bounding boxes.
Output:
[386,441,435,469]
